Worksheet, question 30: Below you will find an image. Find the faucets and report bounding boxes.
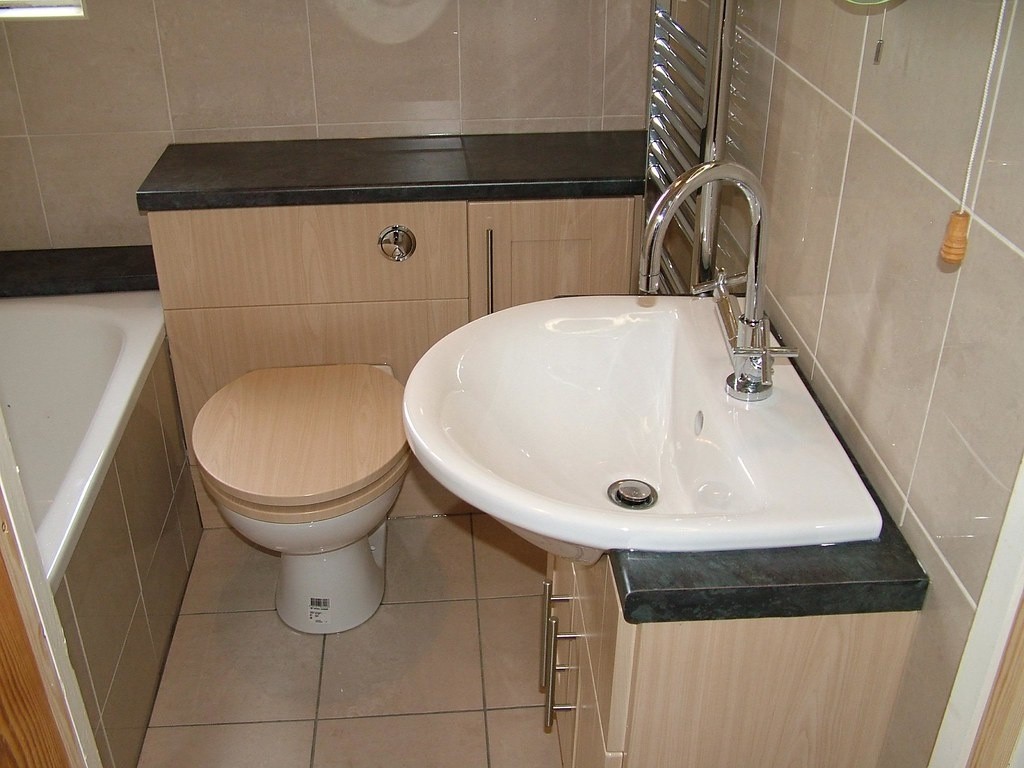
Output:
[637,157,802,402]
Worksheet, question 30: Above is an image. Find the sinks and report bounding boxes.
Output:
[397,291,884,554]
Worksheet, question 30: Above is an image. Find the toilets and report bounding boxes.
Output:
[191,361,416,636]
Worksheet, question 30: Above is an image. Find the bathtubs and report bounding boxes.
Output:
[0,288,209,768]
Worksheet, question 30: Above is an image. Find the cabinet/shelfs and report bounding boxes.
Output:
[136,196,646,466]
[539,554,930,768]
